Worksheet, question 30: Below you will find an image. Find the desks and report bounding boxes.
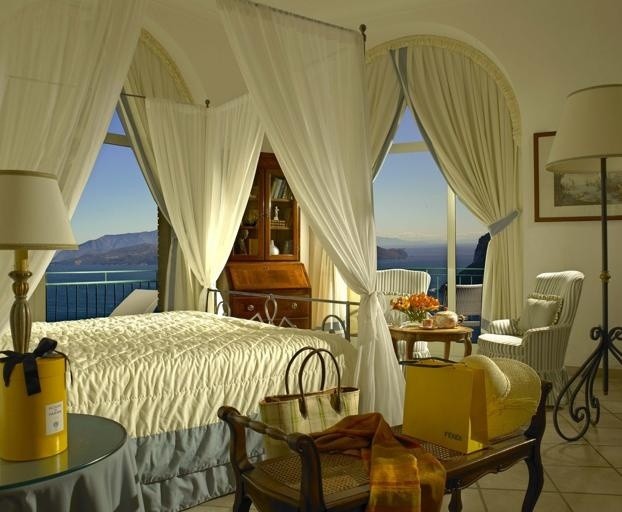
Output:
[389,326,473,362]
[0,412,128,512]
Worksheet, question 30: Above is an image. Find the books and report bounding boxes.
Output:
[271,176,291,199]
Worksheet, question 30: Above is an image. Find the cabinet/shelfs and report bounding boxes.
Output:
[217,152,311,329]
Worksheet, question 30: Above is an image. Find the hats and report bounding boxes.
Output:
[460,354,542,440]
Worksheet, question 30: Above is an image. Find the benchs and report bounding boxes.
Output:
[217,381,553,512]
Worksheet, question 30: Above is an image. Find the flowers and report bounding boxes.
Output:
[390,292,444,322]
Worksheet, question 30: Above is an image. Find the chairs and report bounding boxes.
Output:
[477,271,585,408]
[376,269,431,362]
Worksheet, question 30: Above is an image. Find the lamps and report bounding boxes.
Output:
[545,84,622,442]
[0,169,80,354]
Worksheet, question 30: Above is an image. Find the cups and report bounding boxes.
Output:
[391,310,401,328]
[423,319,437,328]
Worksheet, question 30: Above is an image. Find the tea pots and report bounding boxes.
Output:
[426,306,465,328]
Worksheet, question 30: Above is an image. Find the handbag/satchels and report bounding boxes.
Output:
[399,357,489,454]
[258,347,360,460]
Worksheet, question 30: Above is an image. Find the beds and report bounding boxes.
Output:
[1,289,361,512]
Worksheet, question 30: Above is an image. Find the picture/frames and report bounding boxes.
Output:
[534,132,622,222]
